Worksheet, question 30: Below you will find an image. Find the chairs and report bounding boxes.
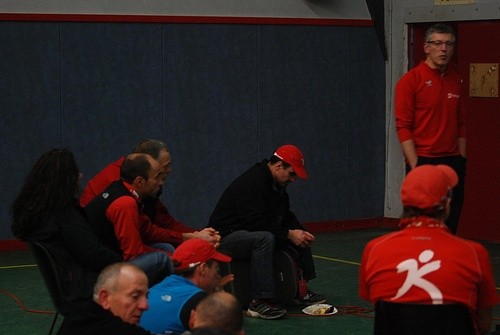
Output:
[373,300,476,335]
[33,239,85,335]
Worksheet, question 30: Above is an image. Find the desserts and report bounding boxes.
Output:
[312,306,333,315]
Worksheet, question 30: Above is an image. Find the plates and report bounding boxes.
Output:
[302,304,338,315]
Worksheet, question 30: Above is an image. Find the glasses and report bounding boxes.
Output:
[428,39,455,48]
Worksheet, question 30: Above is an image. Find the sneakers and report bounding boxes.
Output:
[293,290,327,305]
[246,301,286,319]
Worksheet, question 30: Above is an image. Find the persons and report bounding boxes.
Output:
[159,290,245,335]
[393,21,468,235]
[13,147,177,317]
[80,140,221,245]
[79,153,181,272]
[53,262,159,335]
[199,144,330,320]
[134,238,230,335]
[359,164,500,335]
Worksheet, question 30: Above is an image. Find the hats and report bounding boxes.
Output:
[401,164,458,210]
[172,238,231,270]
[274,144,308,179]
